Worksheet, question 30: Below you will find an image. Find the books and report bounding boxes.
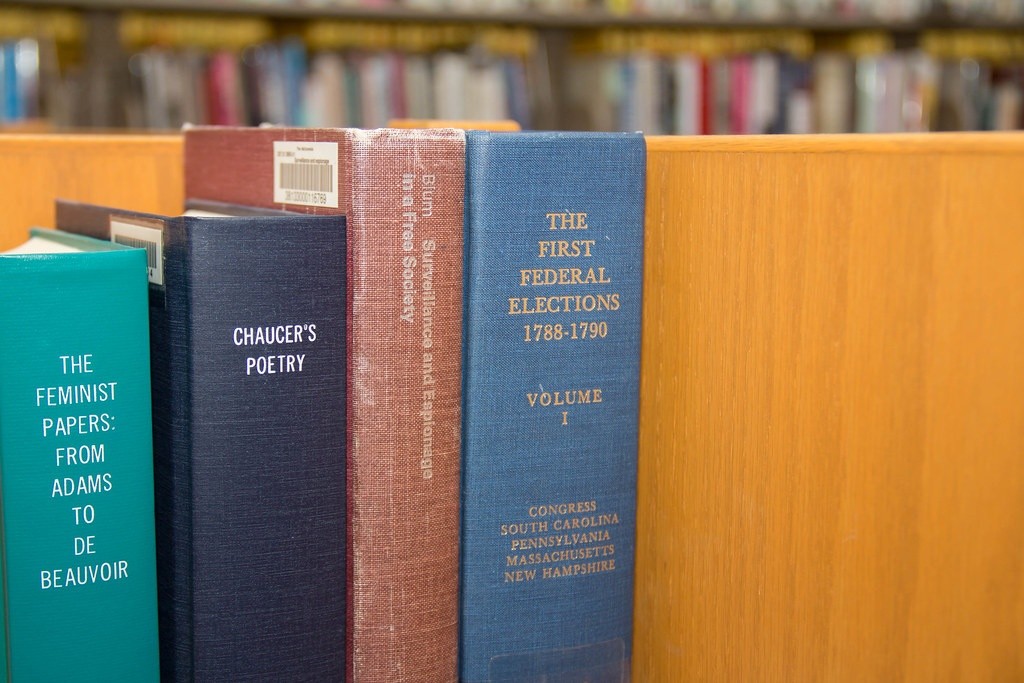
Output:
[460,128,644,683]
[0,1,1024,138]
[184,120,462,683]
[0,196,350,683]
